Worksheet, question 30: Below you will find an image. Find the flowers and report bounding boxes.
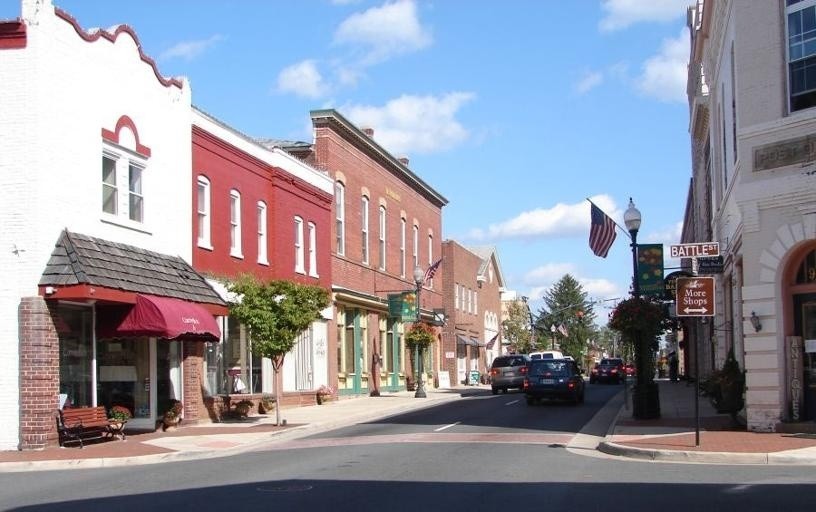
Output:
[162,399,183,422]
[262,391,276,410]
[230,399,254,409]
[108,404,134,420]
[316,384,334,396]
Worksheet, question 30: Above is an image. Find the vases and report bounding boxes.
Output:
[108,418,125,432]
[163,417,180,433]
[234,405,249,415]
[259,402,272,414]
[317,396,334,405]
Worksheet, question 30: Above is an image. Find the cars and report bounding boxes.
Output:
[491,350,586,406]
[589,357,636,385]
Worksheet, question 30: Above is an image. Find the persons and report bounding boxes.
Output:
[670,355,678,383]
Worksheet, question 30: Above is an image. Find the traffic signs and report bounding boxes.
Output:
[675,276,715,317]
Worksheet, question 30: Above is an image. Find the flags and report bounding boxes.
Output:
[589,204,617,259]
[423,260,441,281]
[558,315,569,338]
[486,335,499,351]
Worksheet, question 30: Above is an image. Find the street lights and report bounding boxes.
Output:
[586,334,616,358]
[413,265,427,398]
[624,197,642,299]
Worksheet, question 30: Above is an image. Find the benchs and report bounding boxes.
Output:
[57,406,120,450]
